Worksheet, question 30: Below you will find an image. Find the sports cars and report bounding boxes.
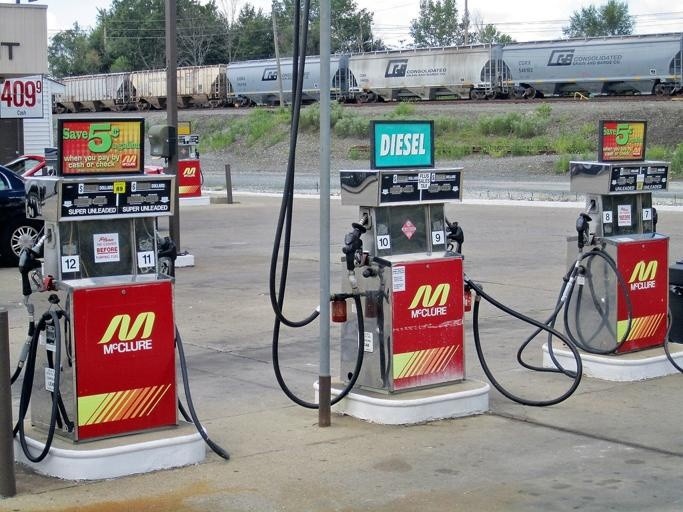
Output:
[2,153,163,178]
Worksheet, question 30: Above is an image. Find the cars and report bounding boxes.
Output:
[0,164,45,266]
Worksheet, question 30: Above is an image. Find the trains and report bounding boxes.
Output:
[50,30,683,114]
